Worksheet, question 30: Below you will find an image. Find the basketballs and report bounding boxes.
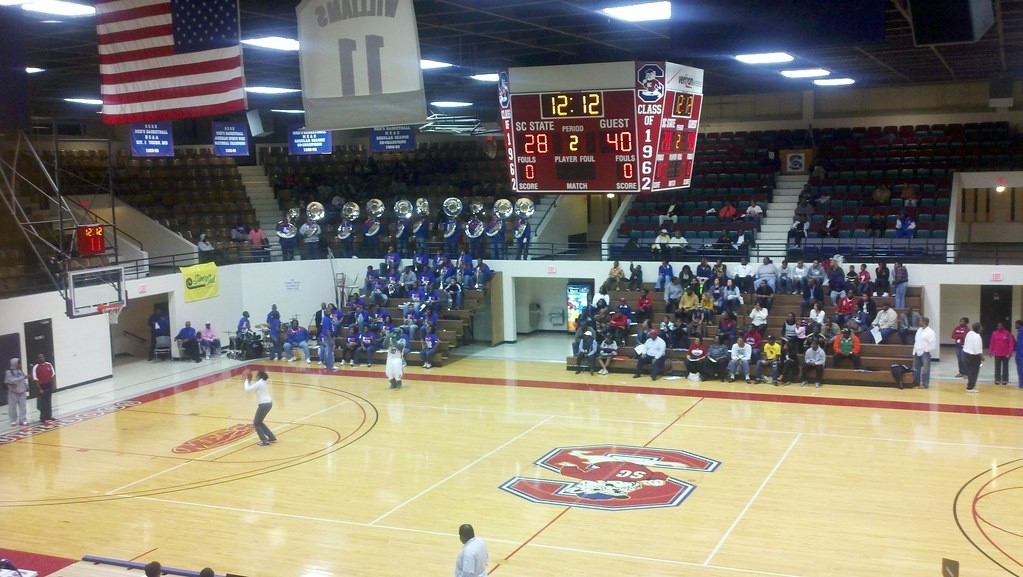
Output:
[242,368,253,382]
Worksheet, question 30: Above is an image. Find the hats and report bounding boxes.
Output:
[841,328,851,334]
[199,233,206,241]
[605,332,613,338]
[650,329,658,335]
[661,229,668,233]
[584,330,592,336]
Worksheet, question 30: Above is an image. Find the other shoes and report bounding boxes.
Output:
[955,374,964,377]
[40,417,56,422]
[912,384,919,389]
[1002,380,1007,385]
[995,380,1000,384]
[268,438,277,441]
[964,374,969,379]
[148,355,153,361]
[20,421,29,426]
[575,367,824,387]
[11,421,17,426]
[919,384,928,388]
[257,442,270,446]
[966,388,979,393]
[196,354,433,389]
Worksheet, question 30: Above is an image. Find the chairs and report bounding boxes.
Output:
[153,335,171,359]
[0,141,537,298]
[610,121,1023,252]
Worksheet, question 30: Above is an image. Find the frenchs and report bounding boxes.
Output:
[364,198,385,236]
[334,202,360,240]
[276,208,300,239]
[464,197,485,238]
[513,197,535,239]
[412,197,430,233]
[306,202,325,238]
[443,196,463,238]
[485,198,513,236]
[393,199,413,239]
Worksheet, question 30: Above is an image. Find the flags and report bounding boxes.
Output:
[77,224,105,256]
[94,0,247,124]
[179,261,220,303]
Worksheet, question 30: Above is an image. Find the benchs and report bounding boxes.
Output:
[309,282,491,367]
[177,339,215,360]
[567,280,925,390]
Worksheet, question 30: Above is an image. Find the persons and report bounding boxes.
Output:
[912,317,936,389]
[174,320,202,363]
[244,370,276,446]
[145,561,161,577]
[248,224,266,262]
[200,568,214,577]
[382,327,407,389]
[147,307,168,361]
[455,524,488,577]
[237,311,250,335]
[952,317,1023,392]
[199,321,221,360]
[315,244,492,369]
[199,234,214,251]
[571,182,917,388]
[4,358,30,426]
[267,304,312,363]
[231,217,249,243]
[31,353,56,423]
[271,155,531,261]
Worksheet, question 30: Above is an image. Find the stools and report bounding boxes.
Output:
[290,348,305,360]
